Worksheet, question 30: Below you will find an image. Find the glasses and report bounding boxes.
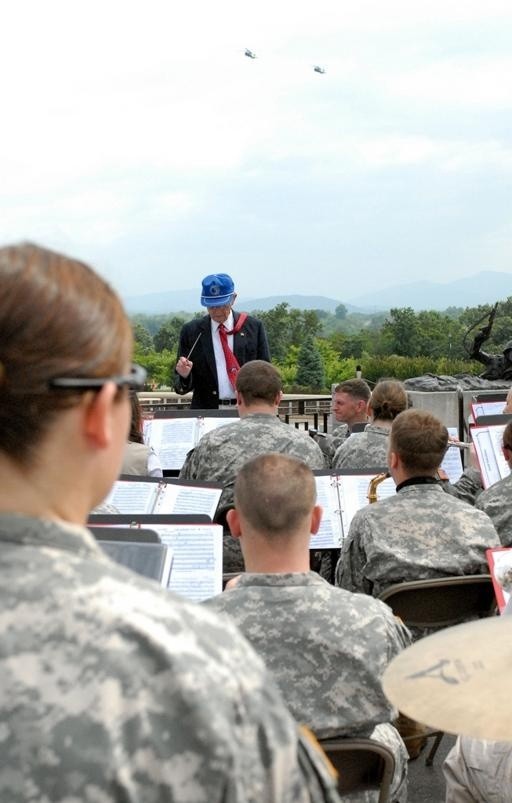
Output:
[42,362,149,398]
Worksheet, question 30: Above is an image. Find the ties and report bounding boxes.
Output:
[218,310,251,393]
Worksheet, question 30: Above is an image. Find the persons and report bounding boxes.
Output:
[330,379,371,453]
[502,385,512,414]
[123,390,165,479]
[176,272,272,408]
[178,361,327,573]
[1,241,346,801]
[335,409,500,644]
[437,736,512,803]
[192,453,428,802]
[332,380,413,468]
[473,420,512,548]
[435,433,487,507]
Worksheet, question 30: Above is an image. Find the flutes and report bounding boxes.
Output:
[448,440,470,449]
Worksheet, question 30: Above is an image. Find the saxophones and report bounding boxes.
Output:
[367,472,393,504]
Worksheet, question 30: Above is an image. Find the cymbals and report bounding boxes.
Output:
[380,614,512,743]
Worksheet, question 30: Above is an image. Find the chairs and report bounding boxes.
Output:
[321,739,397,802]
[375,573,498,770]
[214,504,236,582]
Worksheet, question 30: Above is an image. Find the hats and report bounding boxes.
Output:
[201,272,236,308]
